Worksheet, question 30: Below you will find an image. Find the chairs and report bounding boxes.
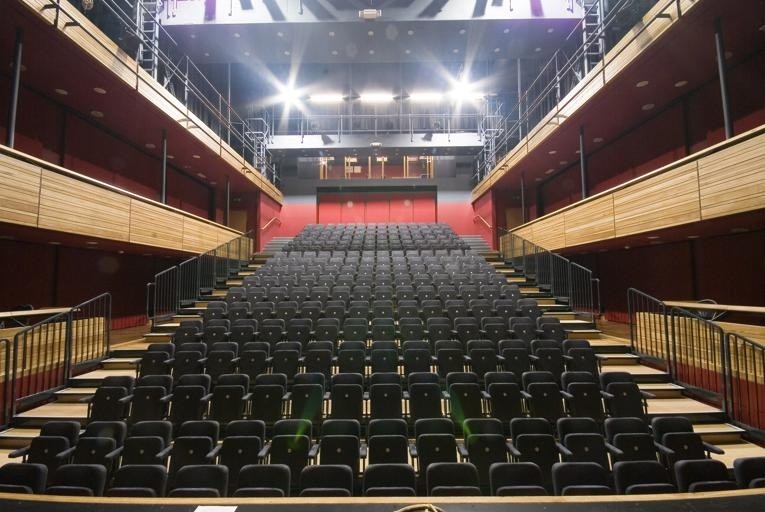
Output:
[282,222,473,248]
